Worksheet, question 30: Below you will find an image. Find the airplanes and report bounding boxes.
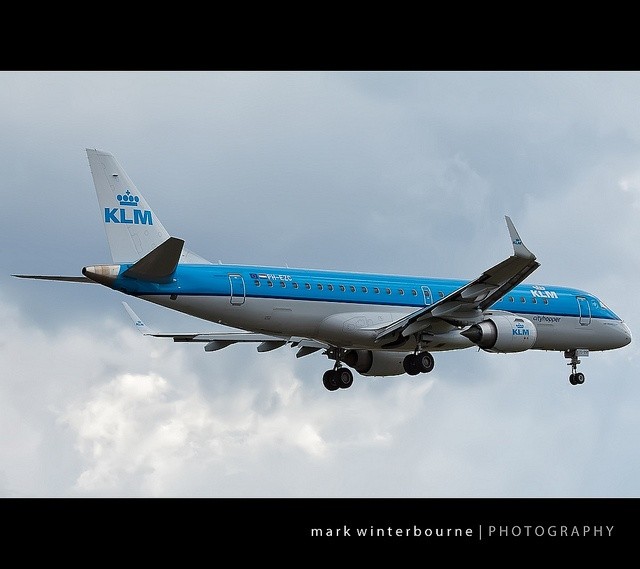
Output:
[12,148,631,391]
